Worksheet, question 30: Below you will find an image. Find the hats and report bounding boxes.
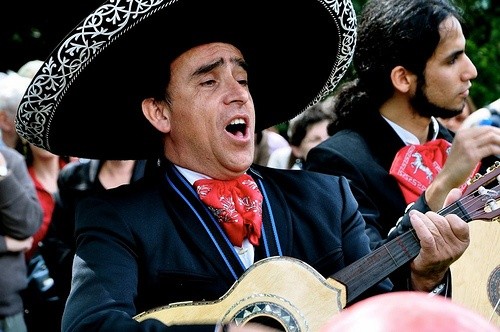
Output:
[15,0,357,160]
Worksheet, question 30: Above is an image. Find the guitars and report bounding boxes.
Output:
[130,160,500,332]
[447,219,500,332]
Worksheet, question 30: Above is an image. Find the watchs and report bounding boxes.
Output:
[407,268,451,297]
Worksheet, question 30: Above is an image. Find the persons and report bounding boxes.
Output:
[0,56,166,331]
[14,0,470,332]
[305,0,500,287]
[253,91,500,200]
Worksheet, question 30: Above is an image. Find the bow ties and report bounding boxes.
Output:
[389,139,481,210]
[193,175,264,248]
